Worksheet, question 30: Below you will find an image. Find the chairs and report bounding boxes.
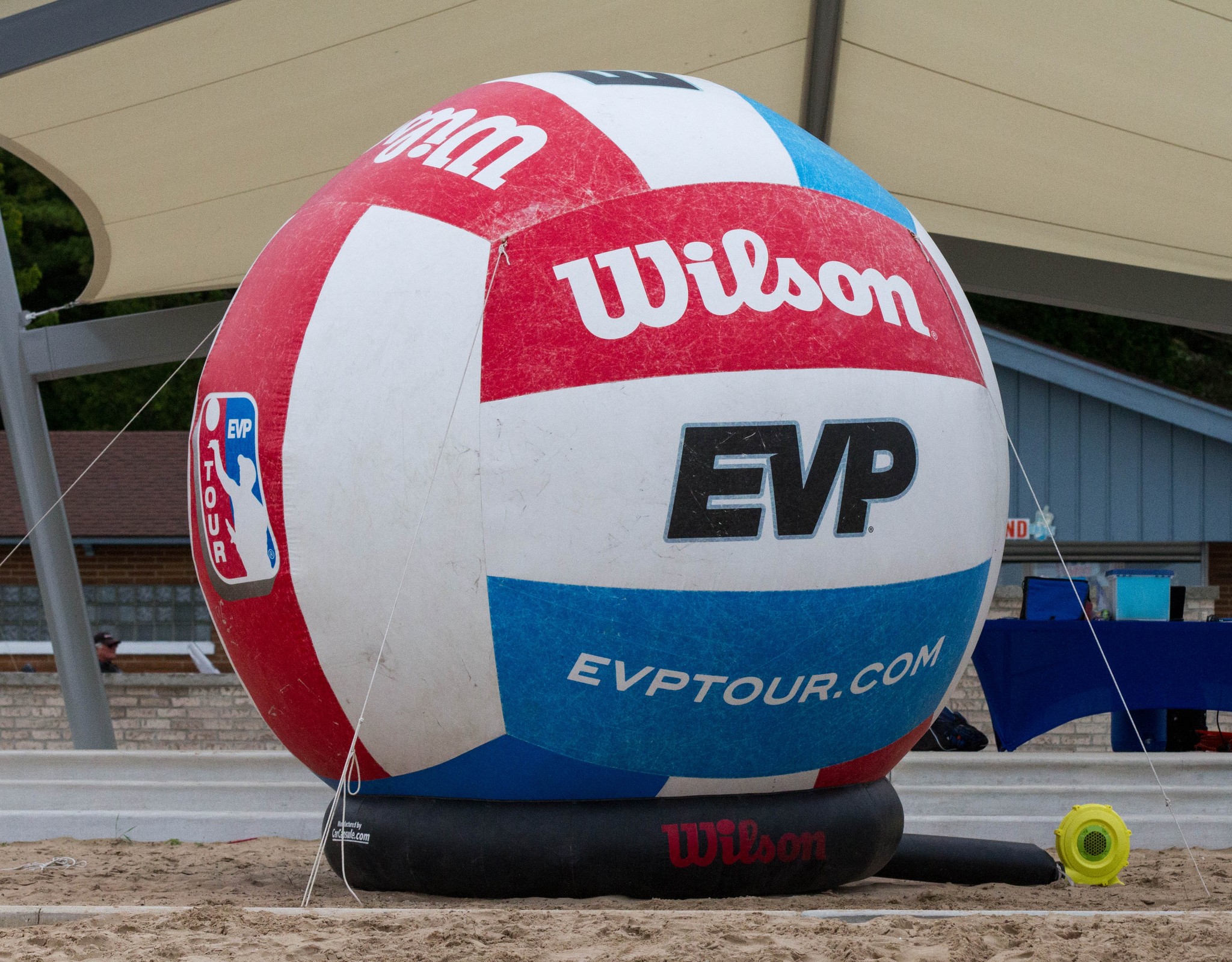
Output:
[1017,574,1089,626]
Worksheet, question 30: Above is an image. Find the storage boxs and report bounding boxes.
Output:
[1105,568,1175,622]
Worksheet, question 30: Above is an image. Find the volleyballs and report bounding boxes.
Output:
[184,67,1009,799]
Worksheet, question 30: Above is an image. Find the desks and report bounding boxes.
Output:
[970,618,1232,753]
